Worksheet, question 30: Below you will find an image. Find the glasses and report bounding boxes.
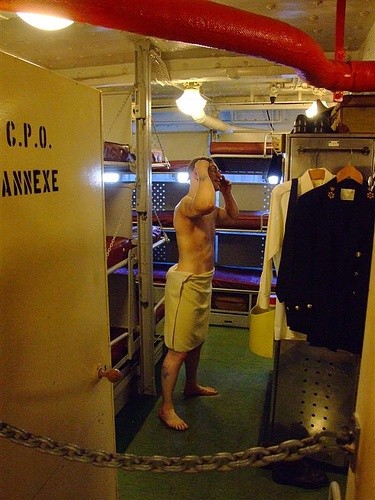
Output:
[207,167,222,174]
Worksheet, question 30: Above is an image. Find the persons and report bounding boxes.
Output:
[158,156,240,432]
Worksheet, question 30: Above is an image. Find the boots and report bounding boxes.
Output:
[270,429,329,488]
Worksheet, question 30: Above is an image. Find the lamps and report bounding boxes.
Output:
[266,150,284,185]
[174,81,207,115]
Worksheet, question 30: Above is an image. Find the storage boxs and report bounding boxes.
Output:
[329,94,375,134]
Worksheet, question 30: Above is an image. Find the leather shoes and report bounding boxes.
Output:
[294,114,312,132]
[312,115,328,132]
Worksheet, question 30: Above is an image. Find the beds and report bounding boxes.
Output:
[103,129,278,415]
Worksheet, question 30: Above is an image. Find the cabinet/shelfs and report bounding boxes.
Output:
[260,134,375,468]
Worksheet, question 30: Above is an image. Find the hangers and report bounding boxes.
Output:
[304,145,368,190]
